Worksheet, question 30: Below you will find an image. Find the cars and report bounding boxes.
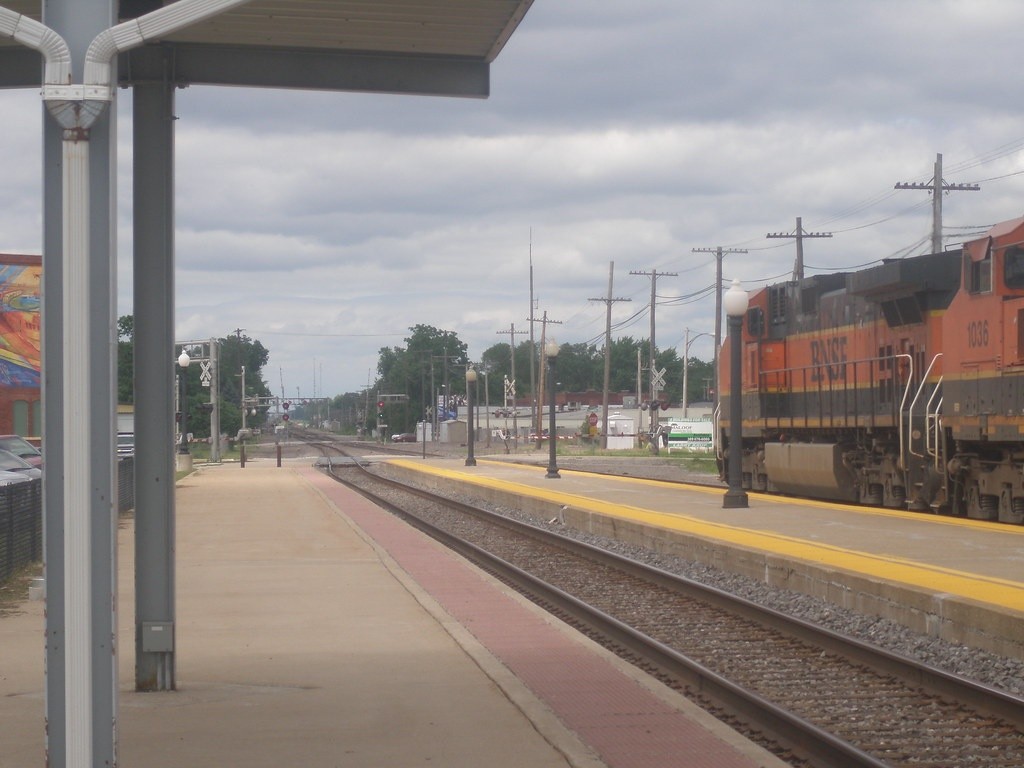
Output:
[391,432,417,443]
[116,433,135,459]
[0,433,44,488]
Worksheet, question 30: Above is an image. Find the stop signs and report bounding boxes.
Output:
[588,413,598,427]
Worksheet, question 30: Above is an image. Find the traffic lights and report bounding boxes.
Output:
[494,410,500,418]
[283,402,289,409]
[282,414,289,421]
[378,413,384,418]
[650,401,661,411]
[378,402,384,406]
[511,411,517,417]
[502,411,509,418]
[661,401,668,410]
[641,403,648,411]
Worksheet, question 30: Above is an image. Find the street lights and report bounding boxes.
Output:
[463,367,478,466]
[177,348,191,455]
[681,329,716,418]
[722,278,749,510]
[544,337,561,479]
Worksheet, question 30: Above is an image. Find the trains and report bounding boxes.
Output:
[709,215,1024,526]
